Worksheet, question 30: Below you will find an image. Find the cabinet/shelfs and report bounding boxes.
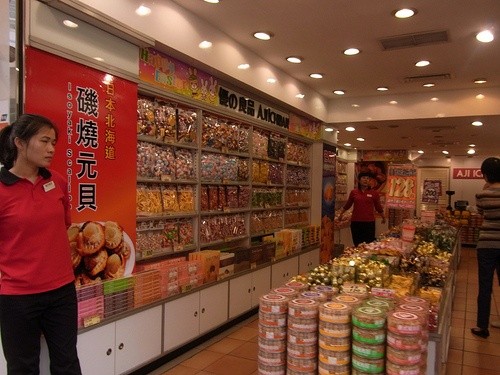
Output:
[134,81,311,256]
[40,244,322,375]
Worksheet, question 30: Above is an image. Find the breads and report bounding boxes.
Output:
[67,220,132,286]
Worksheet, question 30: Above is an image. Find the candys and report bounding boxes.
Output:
[292,214,459,334]
[135,99,310,256]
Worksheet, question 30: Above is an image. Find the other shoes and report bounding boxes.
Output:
[471,327,489,337]
[491,321,500,328]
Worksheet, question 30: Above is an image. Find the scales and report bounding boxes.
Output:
[446,190,469,214]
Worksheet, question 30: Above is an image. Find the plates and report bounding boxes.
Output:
[77,222,134,277]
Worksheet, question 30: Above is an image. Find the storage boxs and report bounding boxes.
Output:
[76,223,321,329]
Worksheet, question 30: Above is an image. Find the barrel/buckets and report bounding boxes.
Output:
[401,225,416,243]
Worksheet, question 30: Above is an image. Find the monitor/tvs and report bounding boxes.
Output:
[455,201,469,211]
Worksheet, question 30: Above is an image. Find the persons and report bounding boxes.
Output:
[0,115,82,375]
[471,157,500,338]
[338,172,385,247]
